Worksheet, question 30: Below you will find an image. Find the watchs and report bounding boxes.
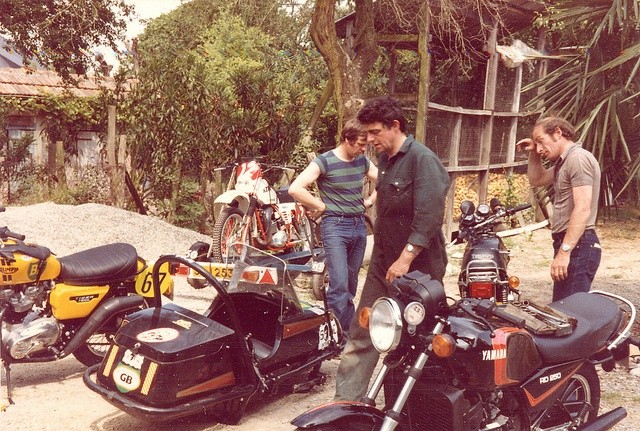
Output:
[405,243,419,256]
[560,243,573,251]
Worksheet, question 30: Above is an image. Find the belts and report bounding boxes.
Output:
[552,229,597,240]
[328,212,363,218]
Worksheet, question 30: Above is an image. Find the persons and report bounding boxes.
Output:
[517,116,601,301]
[333,96,450,402]
[288,119,378,350]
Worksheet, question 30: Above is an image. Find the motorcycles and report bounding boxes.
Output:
[290,270,640,431]
[0,226,173,405]
[446,198,532,305]
[206,124,330,299]
